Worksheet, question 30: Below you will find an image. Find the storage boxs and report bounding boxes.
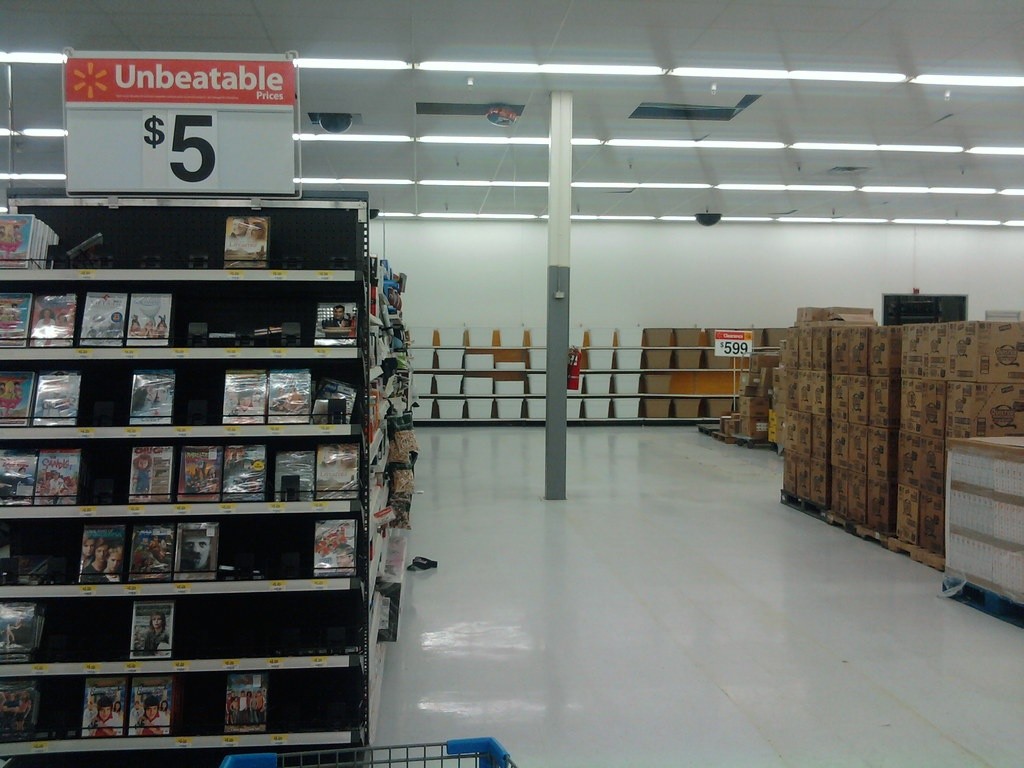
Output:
[409,307,1024,630]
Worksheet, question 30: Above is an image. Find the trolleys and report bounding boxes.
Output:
[218,735,517,768]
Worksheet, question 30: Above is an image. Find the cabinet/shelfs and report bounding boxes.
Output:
[0,192,414,765]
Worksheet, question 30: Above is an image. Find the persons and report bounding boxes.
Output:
[238,467,265,500]
[2,607,26,647]
[318,446,357,471]
[5,464,78,505]
[78,530,211,582]
[82,695,171,737]
[31,307,167,346]
[226,689,267,727]
[0,689,32,731]
[229,216,265,243]
[0,380,307,416]
[143,610,171,657]
[331,543,355,574]
[321,304,356,346]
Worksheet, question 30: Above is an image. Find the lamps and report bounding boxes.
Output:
[695,206,722,226]
[318,112,351,133]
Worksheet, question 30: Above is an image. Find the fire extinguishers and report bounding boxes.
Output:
[567,344,582,390]
